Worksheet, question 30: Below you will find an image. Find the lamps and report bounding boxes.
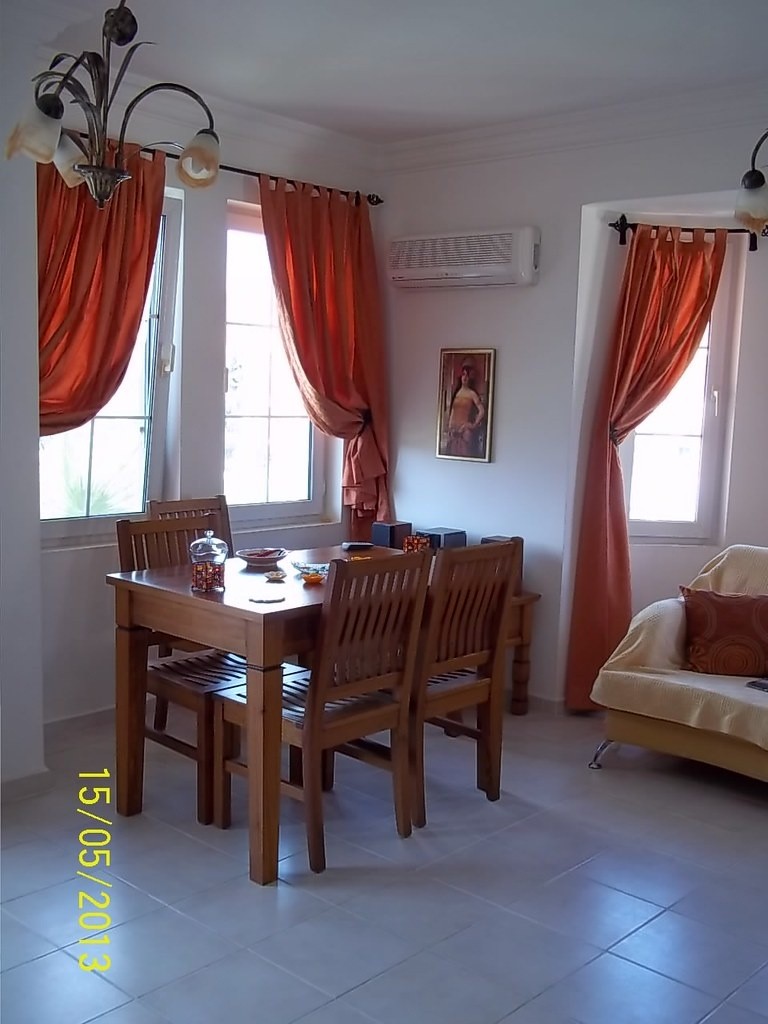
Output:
[736,129,768,237]
[8,0,221,211]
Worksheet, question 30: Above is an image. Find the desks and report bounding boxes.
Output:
[104,542,506,888]
[441,595,542,738]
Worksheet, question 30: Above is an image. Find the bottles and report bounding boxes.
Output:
[190,530,229,593]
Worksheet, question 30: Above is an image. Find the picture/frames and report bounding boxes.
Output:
[436,348,496,463]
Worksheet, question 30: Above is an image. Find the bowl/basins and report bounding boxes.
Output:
[292,560,348,573]
[265,571,288,580]
[302,573,326,584]
[236,547,288,567]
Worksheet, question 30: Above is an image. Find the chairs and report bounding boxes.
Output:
[95,495,520,875]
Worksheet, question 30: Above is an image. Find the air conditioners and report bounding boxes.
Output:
[378,225,542,289]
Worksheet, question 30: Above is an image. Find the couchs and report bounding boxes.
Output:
[590,544,768,786]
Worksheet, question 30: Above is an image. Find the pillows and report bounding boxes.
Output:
[675,586,767,679]
